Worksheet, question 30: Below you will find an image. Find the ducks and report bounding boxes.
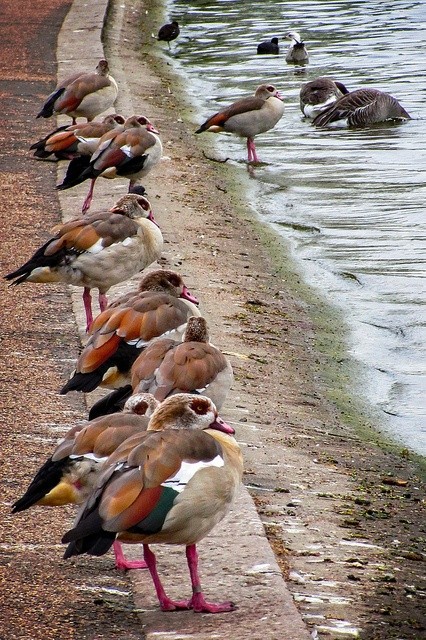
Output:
[311,87,412,128]
[298,77,350,119]
[281,32,308,60]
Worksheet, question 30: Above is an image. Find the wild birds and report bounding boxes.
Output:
[156,21,178,50]
[256,38,279,54]
[34,60,118,125]
[59,269,201,395]
[87,315,233,422]
[59,392,243,614]
[1,193,163,334]
[28,114,125,160]
[9,392,163,572]
[129,186,149,195]
[53,115,161,215]
[194,84,285,165]
